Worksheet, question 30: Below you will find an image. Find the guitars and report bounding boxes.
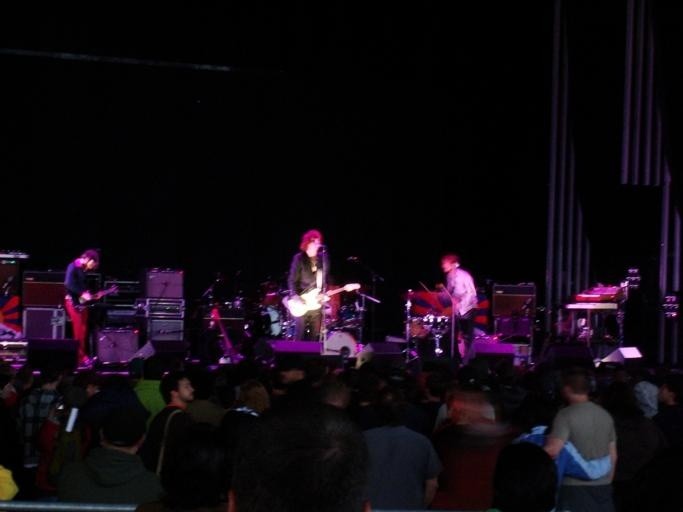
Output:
[72,284,118,311]
[288,283,361,317]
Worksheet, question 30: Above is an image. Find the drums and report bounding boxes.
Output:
[322,331,359,358]
[330,305,364,330]
[256,306,281,337]
[256,282,281,305]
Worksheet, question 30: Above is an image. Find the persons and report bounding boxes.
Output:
[64,250,118,370]
[1,357,683,512]
[288,230,354,342]
[435,254,478,361]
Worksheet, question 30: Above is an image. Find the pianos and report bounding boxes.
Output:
[565,286,625,309]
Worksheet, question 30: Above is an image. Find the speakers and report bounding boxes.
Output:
[146,268,184,298]
[96,327,139,361]
[23,307,66,340]
[493,285,536,344]
